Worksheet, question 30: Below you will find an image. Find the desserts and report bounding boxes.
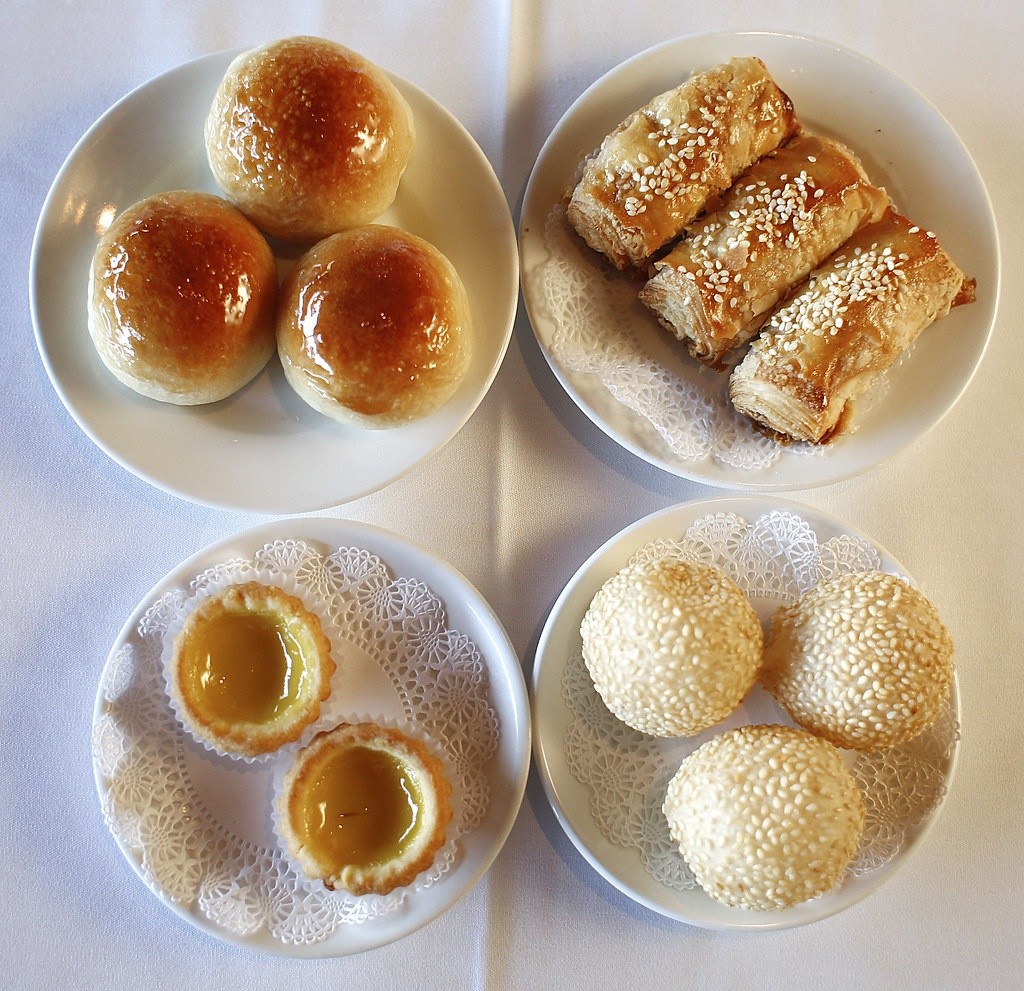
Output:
[270,714,467,897]
[159,567,341,763]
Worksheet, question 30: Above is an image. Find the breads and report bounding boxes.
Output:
[727,205,977,446]
[566,54,797,274]
[637,128,890,368]
[275,223,472,430]
[87,190,284,406]
[200,33,415,248]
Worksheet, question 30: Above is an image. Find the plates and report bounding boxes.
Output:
[531,497,961,933]
[519,33,1000,489]
[94,516,534,957]
[28,48,519,512]
